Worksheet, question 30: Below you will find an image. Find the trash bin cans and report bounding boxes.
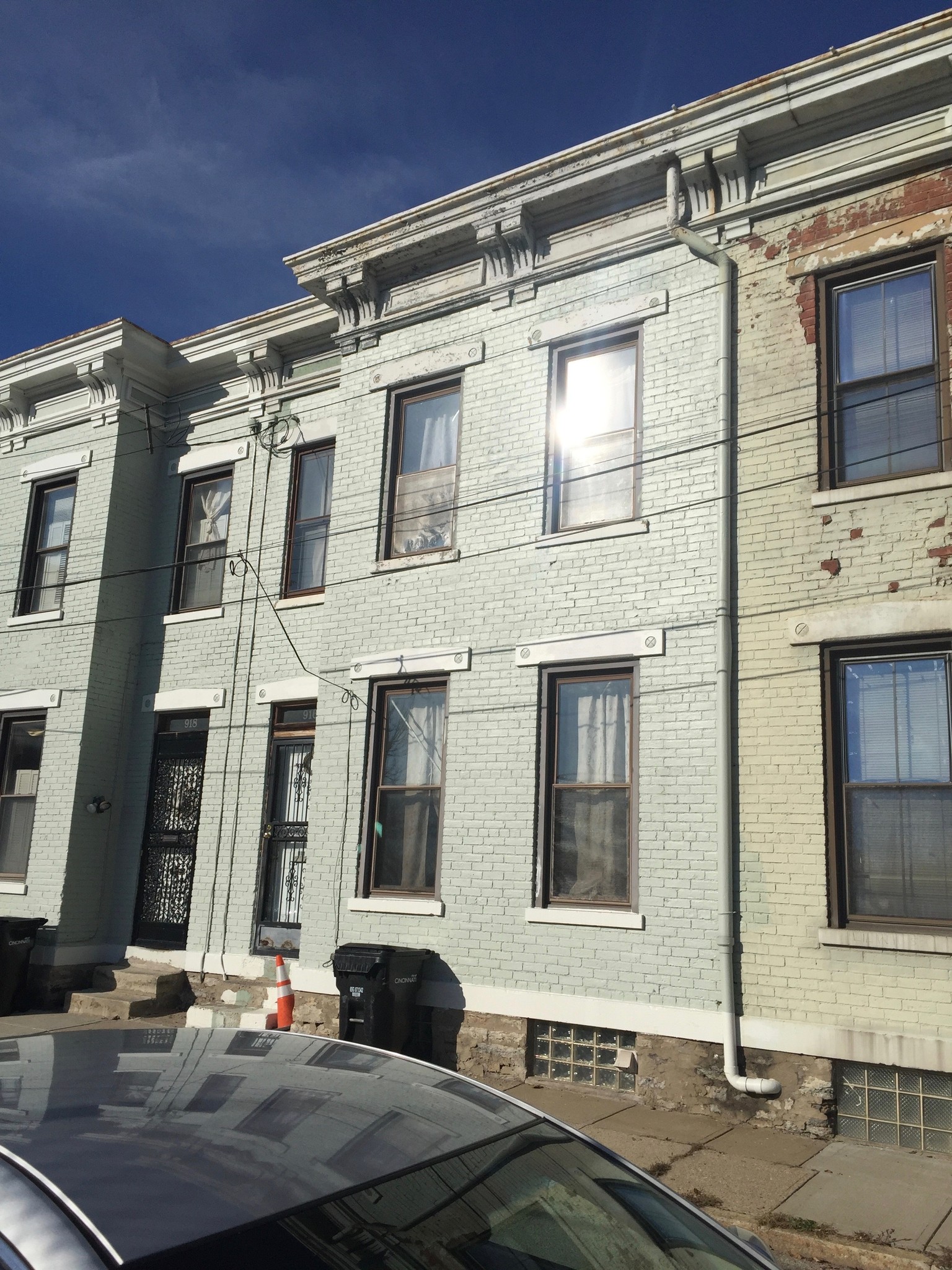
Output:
[330,942,436,1055]
[0,916,48,1017]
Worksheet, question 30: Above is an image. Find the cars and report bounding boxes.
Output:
[0,1026,785,1270]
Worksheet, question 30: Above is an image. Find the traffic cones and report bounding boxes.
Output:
[275,953,293,1030]
